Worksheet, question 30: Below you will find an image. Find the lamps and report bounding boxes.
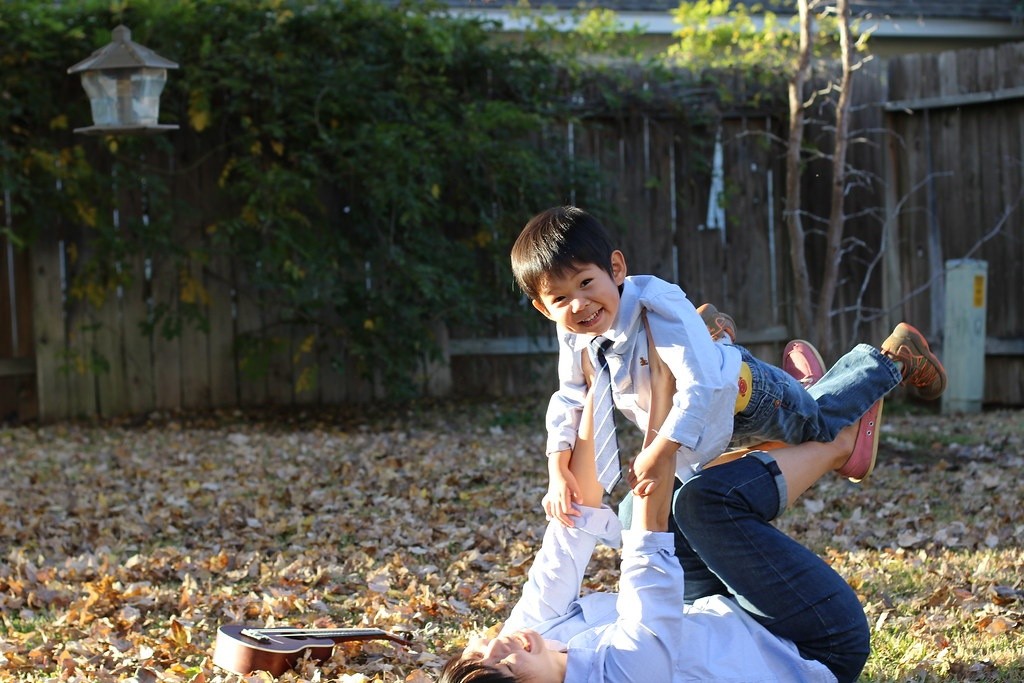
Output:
[63,22,181,139]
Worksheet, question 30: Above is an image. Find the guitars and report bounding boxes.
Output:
[213,621,414,676]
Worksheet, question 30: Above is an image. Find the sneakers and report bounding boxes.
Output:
[880,322,947,400]
[697,303,737,342]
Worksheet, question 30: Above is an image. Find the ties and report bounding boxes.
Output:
[589,336,623,495]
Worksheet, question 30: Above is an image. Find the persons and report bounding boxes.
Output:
[437,340,883,683]
[510,205,945,529]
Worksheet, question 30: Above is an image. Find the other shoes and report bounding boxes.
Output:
[833,395,884,484]
[782,340,827,391]
